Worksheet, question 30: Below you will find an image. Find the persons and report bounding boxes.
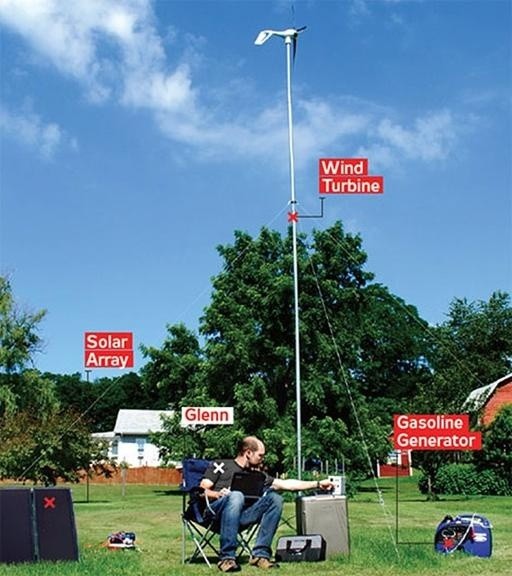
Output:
[198,434,335,573]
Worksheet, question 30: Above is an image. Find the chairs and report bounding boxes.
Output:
[180,460,262,569]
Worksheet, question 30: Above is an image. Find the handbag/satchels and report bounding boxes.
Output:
[275,534,327,562]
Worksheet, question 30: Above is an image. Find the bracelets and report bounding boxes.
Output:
[317,481,320,488]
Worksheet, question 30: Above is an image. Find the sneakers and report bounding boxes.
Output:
[250,557,280,570]
[217,559,241,572]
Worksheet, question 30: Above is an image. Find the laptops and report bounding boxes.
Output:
[230,472,266,499]
